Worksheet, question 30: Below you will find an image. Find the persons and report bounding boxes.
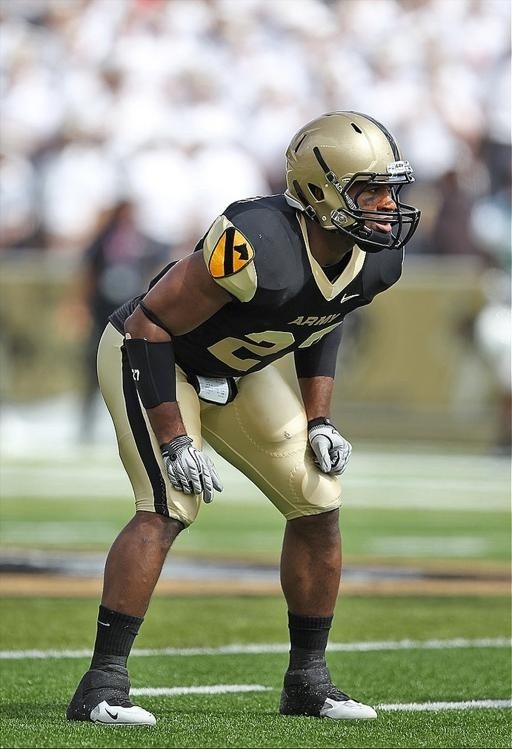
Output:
[65,110,423,726]
[70,201,176,449]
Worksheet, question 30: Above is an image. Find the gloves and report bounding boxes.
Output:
[308,416,352,475]
[160,434,223,504]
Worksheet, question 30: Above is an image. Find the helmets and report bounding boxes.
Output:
[284,110,422,253]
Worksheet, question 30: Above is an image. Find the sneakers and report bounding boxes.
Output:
[66,664,157,727]
[279,658,377,720]
